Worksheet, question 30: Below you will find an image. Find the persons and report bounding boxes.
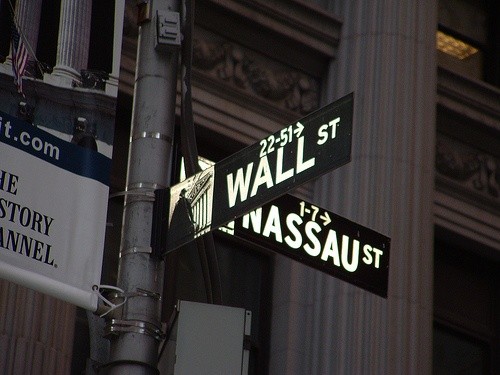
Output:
[171,188,196,248]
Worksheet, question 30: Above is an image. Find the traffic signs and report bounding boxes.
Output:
[164,89,358,253]
[179,154,392,299]
